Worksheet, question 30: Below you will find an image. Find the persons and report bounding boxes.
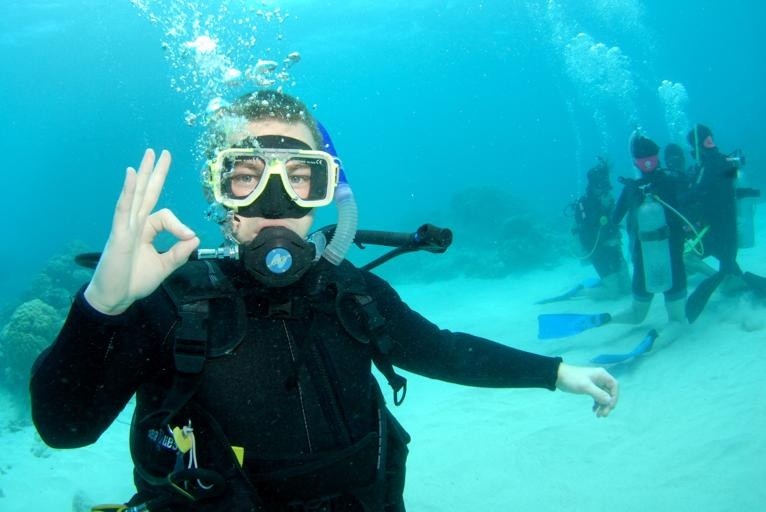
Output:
[560,122,766,359]
[28,89,621,511]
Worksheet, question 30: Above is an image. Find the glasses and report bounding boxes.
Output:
[207,149,338,208]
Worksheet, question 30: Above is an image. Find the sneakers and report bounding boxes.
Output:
[565,286,581,301]
[699,272,727,291]
[578,313,611,332]
[635,329,657,353]
[740,269,757,285]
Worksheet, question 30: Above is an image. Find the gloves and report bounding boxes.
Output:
[555,362,620,416]
[85,145,200,315]
